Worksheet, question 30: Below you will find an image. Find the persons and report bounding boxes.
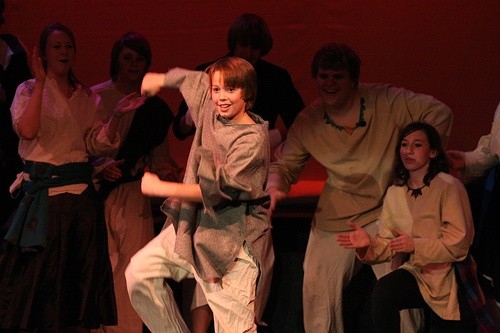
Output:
[1,31,31,269]
[336,121,474,333]
[125,57,270,333]
[260,42,454,333]
[447,102,500,308]
[8,23,150,332]
[169,13,307,332]
[87,37,175,333]
